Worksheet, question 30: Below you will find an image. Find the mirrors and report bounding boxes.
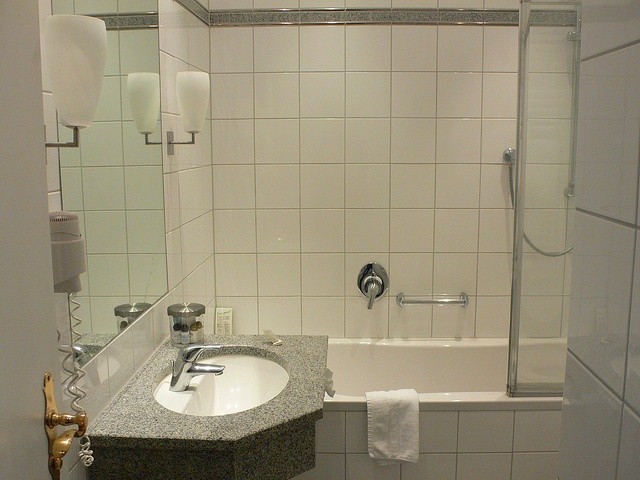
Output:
[38,1,169,384]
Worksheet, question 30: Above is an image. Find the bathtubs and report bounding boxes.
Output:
[325,338,567,409]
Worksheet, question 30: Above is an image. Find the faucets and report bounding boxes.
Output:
[171,343,224,391]
[59,345,91,367]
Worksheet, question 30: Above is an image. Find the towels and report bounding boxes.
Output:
[365,388,420,465]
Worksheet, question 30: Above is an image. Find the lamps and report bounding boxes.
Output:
[127,72,161,144]
[44,14,107,149]
[167,70,211,155]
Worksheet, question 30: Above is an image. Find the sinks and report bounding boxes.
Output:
[151,346,289,416]
